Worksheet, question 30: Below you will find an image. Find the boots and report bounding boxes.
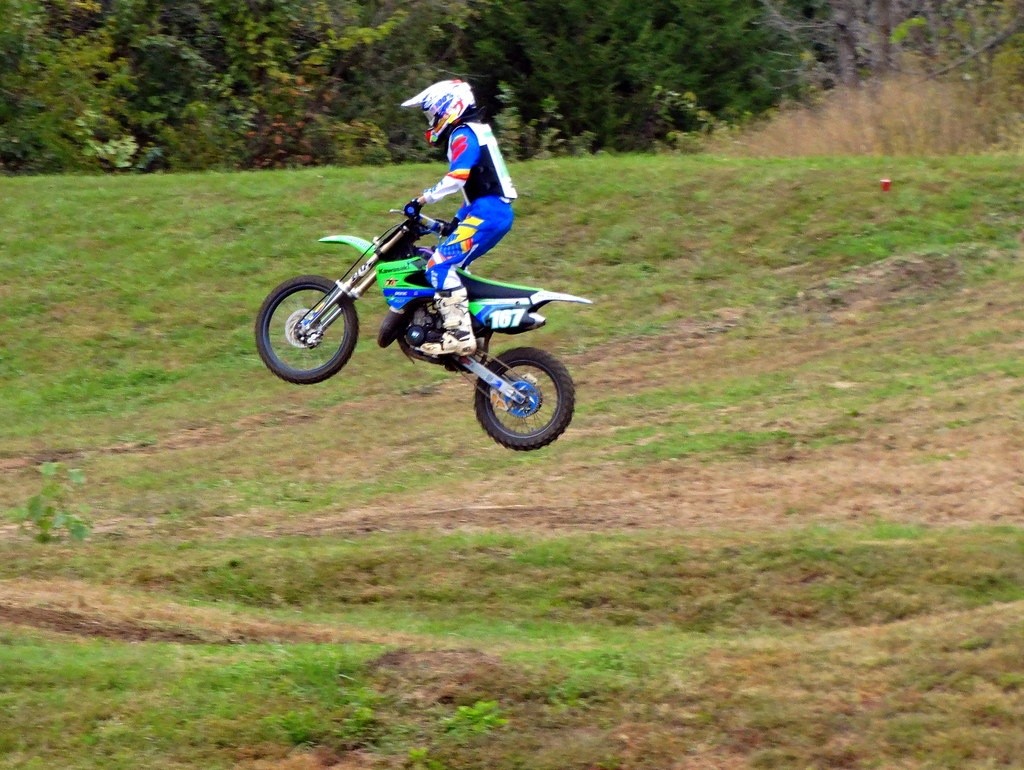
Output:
[418,287,476,356]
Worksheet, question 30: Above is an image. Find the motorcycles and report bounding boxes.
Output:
[253,196,594,453]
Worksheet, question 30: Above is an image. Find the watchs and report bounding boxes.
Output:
[410,197,418,203]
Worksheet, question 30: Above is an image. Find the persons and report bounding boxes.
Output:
[399,77,519,358]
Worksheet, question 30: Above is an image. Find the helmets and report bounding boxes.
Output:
[400,78,488,147]
